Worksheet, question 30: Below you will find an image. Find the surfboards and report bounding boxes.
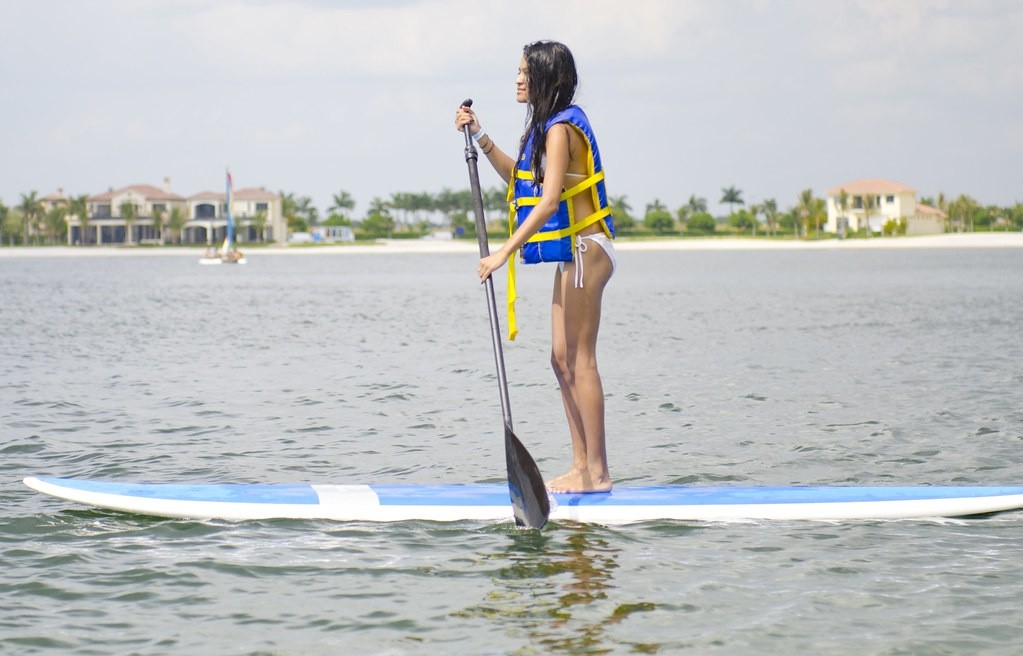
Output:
[23,475,1023,519]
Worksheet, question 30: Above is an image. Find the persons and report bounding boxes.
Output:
[455,41,618,493]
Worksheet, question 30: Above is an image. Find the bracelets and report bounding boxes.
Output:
[484,141,494,154]
[481,135,489,149]
[473,128,485,140]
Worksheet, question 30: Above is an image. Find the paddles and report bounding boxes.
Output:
[459,98,551,530]
